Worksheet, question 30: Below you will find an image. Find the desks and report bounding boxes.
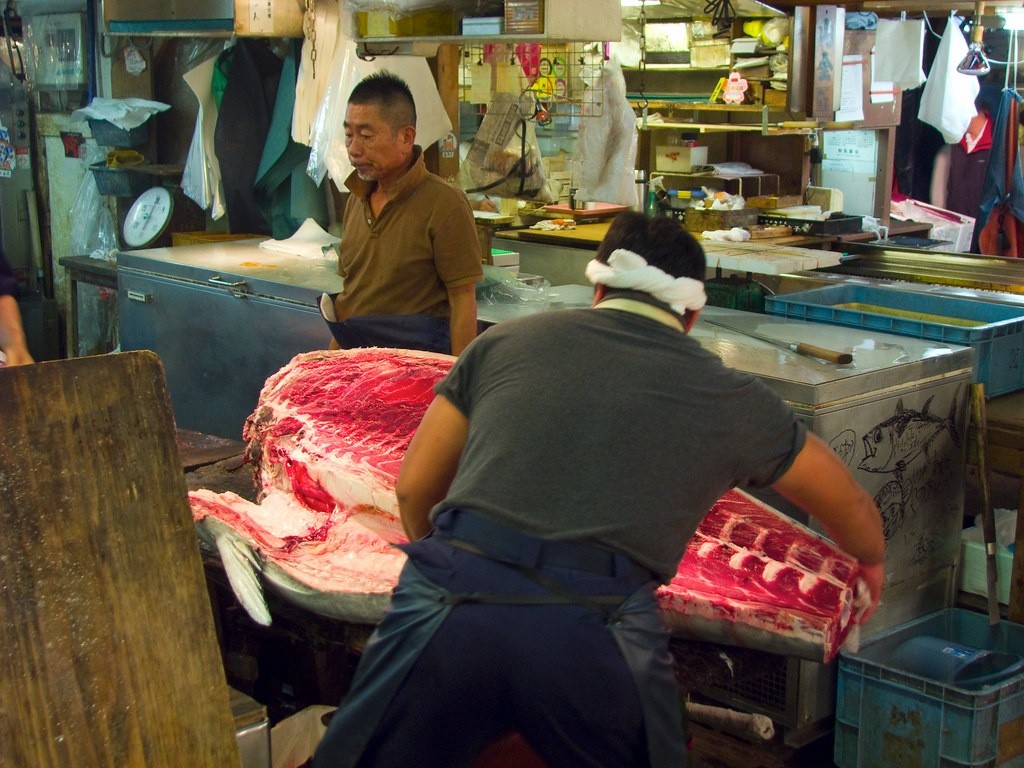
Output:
[650,171,781,209]
[60,254,116,357]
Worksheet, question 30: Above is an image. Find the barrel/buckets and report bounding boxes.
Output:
[886,633,1024,691]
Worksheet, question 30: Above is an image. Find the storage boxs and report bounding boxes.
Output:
[746,194,803,209]
[654,145,709,172]
[909,199,977,253]
[667,188,705,209]
[688,37,786,107]
[765,282,1024,400]
[685,210,758,235]
[705,281,763,309]
[504,0,545,34]
[960,526,1013,604]
[827,608,1024,768]
[462,17,499,37]
[356,12,396,37]
[758,216,866,237]
[893,204,958,252]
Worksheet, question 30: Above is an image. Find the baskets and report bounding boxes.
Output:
[88,159,151,198]
[84,115,149,148]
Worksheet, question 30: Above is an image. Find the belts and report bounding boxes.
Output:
[433,510,654,584]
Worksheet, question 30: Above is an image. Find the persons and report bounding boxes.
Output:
[467,194,498,213]
[328,68,483,358]
[294,208,885,768]
[0,249,35,368]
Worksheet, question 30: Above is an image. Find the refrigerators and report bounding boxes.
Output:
[475,284,976,751]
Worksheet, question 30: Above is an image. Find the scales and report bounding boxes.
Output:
[117,162,206,251]
